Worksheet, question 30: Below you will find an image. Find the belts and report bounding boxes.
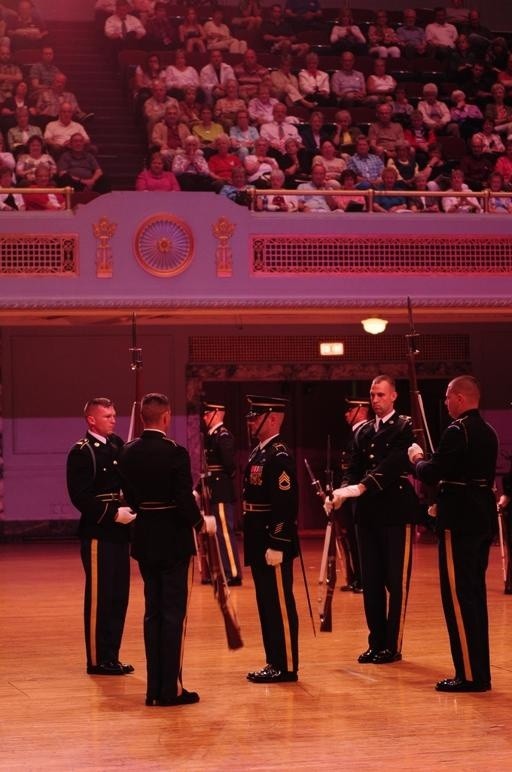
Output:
[208,465,222,471]
[364,469,407,479]
[439,478,488,487]
[97,493,120,502]
[139,501,178,510]
[244,504,272,512]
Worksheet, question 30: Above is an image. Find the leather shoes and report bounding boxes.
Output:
[358,649,402,663]
[226,578,241,586]
[435,679,491,693]
[87,661,134,675]
[247,664,297,682]
[145,688,200,705]
[341,585,363,593]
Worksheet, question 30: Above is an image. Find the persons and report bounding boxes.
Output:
[239,394,300,684]
[2,1,103,211]
[334,396,370,595]
[407,375,498,692]
[65,396,135,676]
[191,399,243,586]
[119,394,200,707]
[107,1,510,213]
[323,375,414,664]
[496,451,511,594]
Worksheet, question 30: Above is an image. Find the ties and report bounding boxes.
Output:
[106,439,115,448]
[379,420,383,430]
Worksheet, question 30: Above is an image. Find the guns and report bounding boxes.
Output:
[128,311,144,438]
[193,432,243,650]
[305,434,358,631]
[405,297,428,467]
[492,479,512,593]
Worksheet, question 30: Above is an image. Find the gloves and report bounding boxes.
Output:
[192,489,201,502]
[204,514,217,534]
[115,506,138,525]
[429,503,438,517]
[265,549,284,566]
[407,442,424,464]
[323,485,360,516]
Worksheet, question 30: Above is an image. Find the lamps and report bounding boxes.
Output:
[319,342,344,357]
[361,313,390,335]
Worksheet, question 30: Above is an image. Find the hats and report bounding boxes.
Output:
[200,401,225,415]
[244,395,289,419]
[345,397,370,411]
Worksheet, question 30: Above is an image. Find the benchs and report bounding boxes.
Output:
[3,1,512,208]
